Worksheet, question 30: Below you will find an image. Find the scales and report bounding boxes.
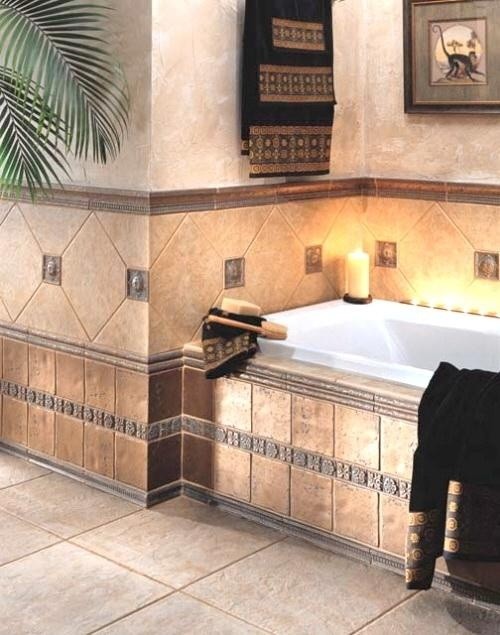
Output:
[255,298,500,389]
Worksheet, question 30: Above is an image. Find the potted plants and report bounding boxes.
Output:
[400,1,500,114]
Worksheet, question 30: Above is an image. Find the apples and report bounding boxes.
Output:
[347,249,370,301]
